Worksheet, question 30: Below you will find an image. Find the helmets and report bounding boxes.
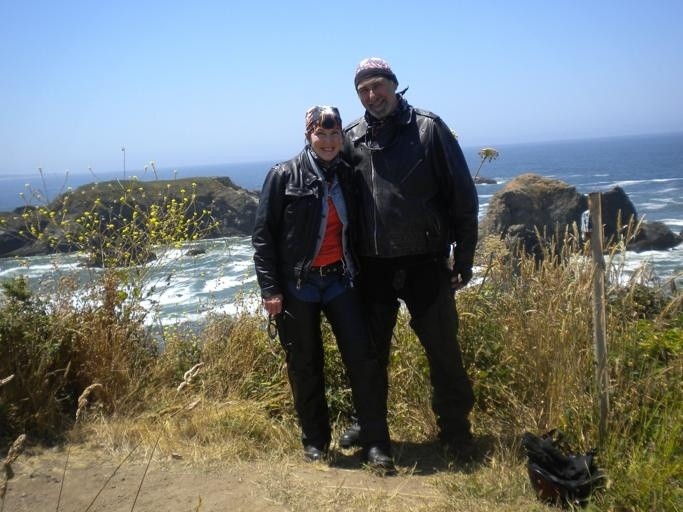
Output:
[520,427,606,509]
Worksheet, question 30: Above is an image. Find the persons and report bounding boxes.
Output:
[250,104,400,478]
[333,55,483,470]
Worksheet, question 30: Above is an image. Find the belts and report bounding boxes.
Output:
[310,260,344,278]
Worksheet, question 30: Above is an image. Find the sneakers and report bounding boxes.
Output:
[302,444,329,463]
[440,438,477,468]
[339,423,364,447]
[363,443,395,472]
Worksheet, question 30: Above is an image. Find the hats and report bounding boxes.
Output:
[354,56,400,88]
